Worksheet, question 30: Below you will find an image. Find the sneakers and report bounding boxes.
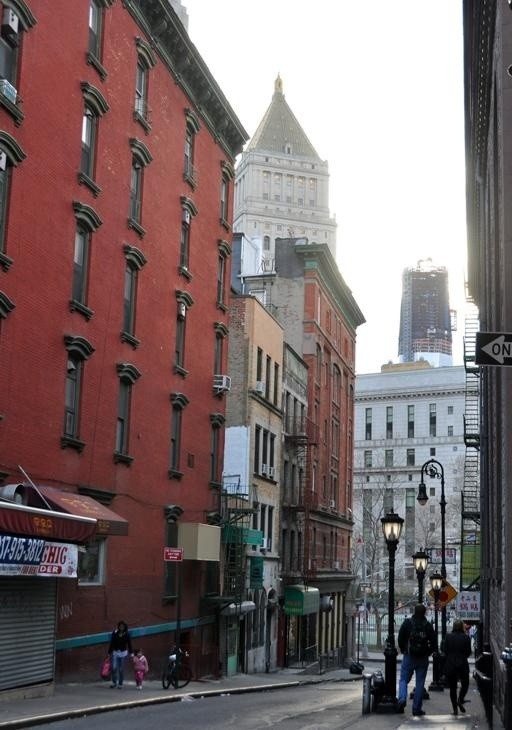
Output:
[109,682,123,689]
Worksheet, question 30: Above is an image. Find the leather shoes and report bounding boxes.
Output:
[396,702,425,716]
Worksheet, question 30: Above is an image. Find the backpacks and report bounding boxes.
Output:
[405,618,432,657]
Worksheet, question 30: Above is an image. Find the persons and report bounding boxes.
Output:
[396,603,438,716]
[440,620,476,715]
[130,648,149,690]
[107,620,134,689]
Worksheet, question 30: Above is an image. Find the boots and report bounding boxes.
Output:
[450,690,467,715]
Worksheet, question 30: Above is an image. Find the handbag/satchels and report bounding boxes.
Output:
[100,654,112,681]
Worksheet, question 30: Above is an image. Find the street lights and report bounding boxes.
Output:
[381,507,404,698]
[412,458,448,692]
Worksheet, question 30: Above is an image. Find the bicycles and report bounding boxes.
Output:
[162,649,191,690]
